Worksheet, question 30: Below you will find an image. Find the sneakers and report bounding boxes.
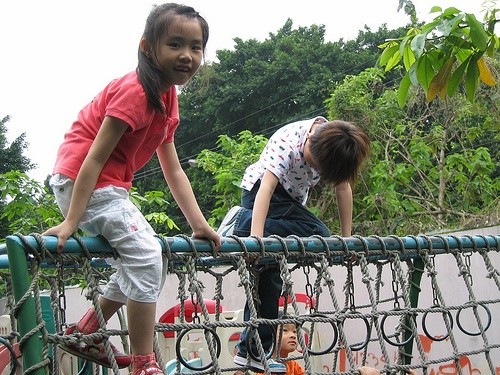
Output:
[231,341,286,374]
[58,322,130,369]
[216,204,244,237]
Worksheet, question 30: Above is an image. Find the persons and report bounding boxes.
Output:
[39,3,222,375]
[216,115,371,373]
[234,310,418,375]
